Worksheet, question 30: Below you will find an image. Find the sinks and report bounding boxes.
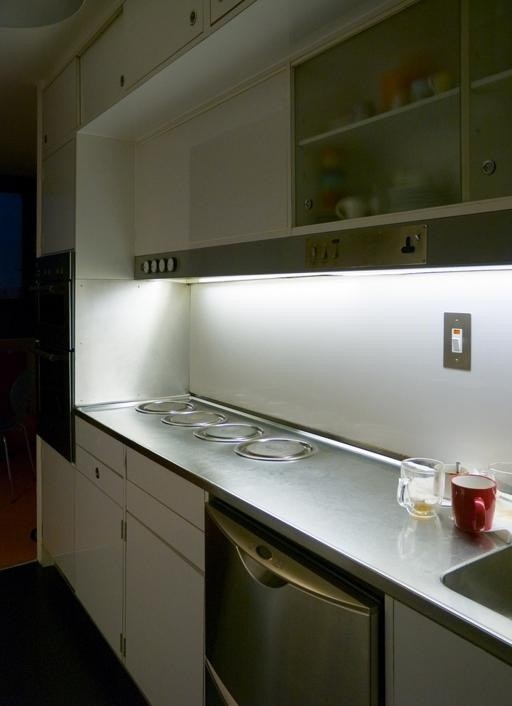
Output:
[439,541,512,622]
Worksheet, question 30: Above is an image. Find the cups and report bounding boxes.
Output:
[450,473,498,537]
[433,462,468,507]
[396,457,445,520]
[426,73,457,96]
[335,197,370,221]
[493,460,512,527]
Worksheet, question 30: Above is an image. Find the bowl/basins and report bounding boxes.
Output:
[370,65,427,113]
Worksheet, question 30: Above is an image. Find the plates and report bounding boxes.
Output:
[388,165,455,216]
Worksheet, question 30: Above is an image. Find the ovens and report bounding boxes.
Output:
[26,248,74,466]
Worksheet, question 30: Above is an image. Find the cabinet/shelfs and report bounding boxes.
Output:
[35,407,512,706]
[36,0,512,282]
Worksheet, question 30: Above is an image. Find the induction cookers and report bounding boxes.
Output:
[133,400,318,463]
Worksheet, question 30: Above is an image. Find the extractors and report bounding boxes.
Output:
[131,223,429,285]
